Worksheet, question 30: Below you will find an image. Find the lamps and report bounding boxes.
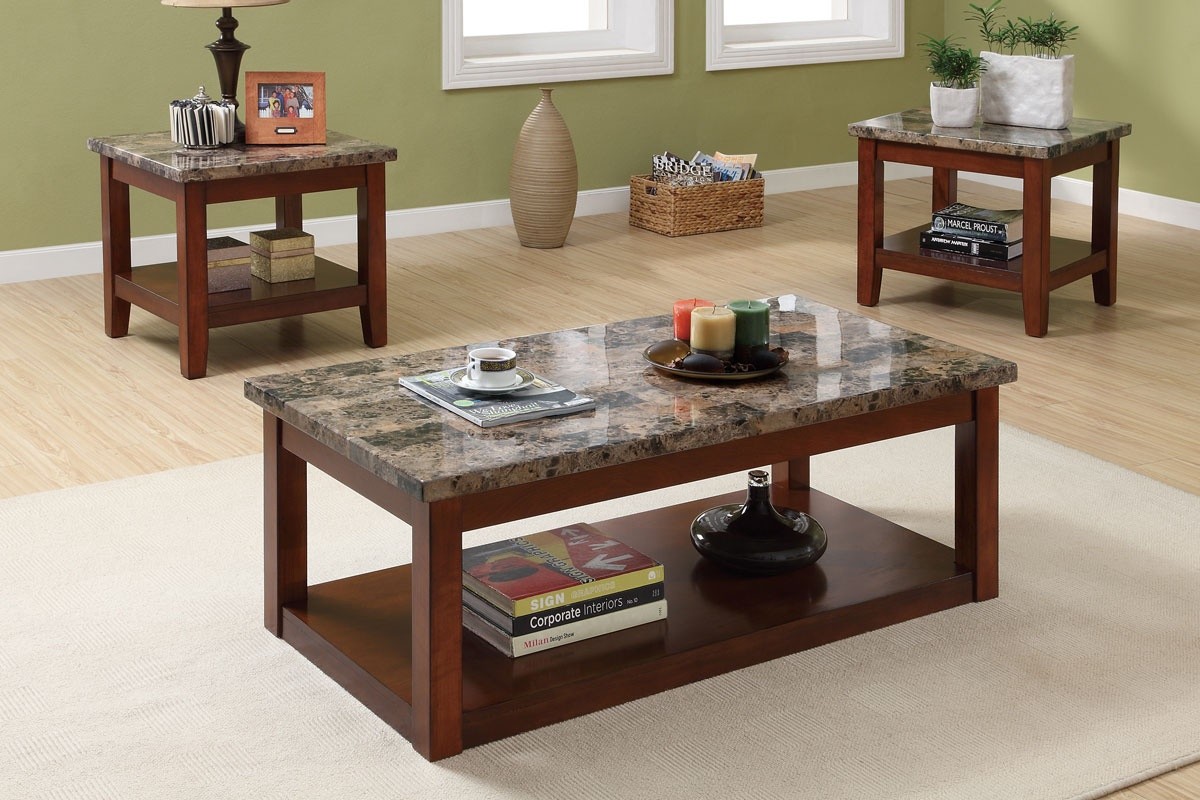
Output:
[161,1,291,145]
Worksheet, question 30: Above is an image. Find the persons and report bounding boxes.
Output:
[268,85,302,117]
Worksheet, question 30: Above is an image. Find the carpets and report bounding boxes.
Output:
[0,420,1200,800]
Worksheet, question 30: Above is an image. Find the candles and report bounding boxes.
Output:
[673,298,713,341]
[690,306,736,353]
[728,300,773,350]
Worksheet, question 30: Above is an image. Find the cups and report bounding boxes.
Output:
[467,348,517,388]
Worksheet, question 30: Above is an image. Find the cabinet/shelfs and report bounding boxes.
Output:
[87,130,397,382]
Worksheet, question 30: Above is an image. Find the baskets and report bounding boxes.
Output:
[629,175,764,237]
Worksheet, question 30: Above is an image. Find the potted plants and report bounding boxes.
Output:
[964,0,1080,129]
[914,32,990,128]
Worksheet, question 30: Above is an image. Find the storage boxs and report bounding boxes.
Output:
[249,228,315,282]
[628,174,765,238]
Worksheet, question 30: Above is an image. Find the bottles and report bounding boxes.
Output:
[512,90,577,248]
[690,471,828,572]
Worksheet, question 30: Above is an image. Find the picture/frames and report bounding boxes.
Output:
[244,72,327,146]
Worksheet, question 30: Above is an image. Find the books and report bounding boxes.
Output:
[919,202,1023,261]
[649,149,758,195]
[462,522,667,660]
[398,364,596,427]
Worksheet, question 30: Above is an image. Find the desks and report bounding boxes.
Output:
[846,107,1127,335]
[241,295,1020,765]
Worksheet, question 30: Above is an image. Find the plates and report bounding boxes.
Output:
[449,367,536,396]
[640,339,791,379]
